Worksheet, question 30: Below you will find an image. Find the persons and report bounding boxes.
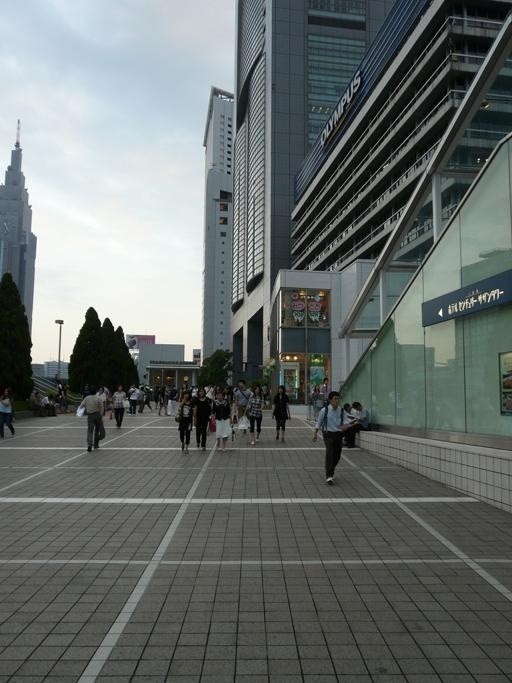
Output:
[348,402,370,448]
[272,385,292,444]
[1,378,271,455]
[320,377,328,408]
[342,403,357,447]
[313,391,351,485]
[312,384,320,423]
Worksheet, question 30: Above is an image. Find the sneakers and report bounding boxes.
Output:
[326,476,334,483]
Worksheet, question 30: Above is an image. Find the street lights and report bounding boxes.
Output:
[55,320,64,381]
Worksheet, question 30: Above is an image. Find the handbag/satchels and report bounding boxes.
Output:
[209,414,216,431]
[174,411,182,422]
